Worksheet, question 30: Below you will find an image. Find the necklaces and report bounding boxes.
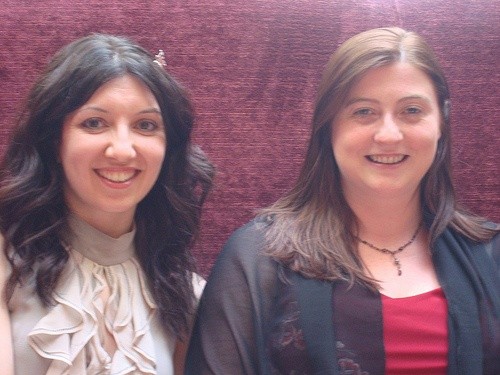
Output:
[342,215,424,277]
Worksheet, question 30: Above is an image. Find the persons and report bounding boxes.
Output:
[0,30,214,375]
[183,26,500,375]
